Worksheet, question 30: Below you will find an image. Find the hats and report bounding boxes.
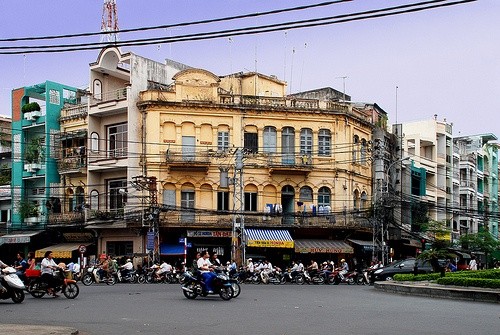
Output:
[248,258,252,260]
[341,259,345,262]
[100,254,107,258]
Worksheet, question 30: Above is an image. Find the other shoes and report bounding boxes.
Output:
[208,290,214,293]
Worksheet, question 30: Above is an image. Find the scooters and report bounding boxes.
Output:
[0,264,26,304]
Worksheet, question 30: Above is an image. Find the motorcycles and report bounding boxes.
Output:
[28,265,80,299]
[13,259,375,300]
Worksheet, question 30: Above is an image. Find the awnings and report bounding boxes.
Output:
[0,229,46,245]
[348,238,377,247]
[456,251,471,258]
[244,228,295,249]
[294,237,354,254]
[35,241,95,259]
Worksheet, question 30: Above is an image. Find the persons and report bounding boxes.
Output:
[339,258,349,281]
[147,259,172,280]
[446,260,456,272]
[192,250,237,293]
[240,257,282,283]
[0,259,10,293]
[287,258,333,280]
[100,250,133,282]
[469,255,478,271]
[369,260,383,286]
[14,251,40,289]
[40,251,80,297]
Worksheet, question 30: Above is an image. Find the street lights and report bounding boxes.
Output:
[385,155,411,265]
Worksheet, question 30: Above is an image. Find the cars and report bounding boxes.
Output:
[373,257,437,281]
